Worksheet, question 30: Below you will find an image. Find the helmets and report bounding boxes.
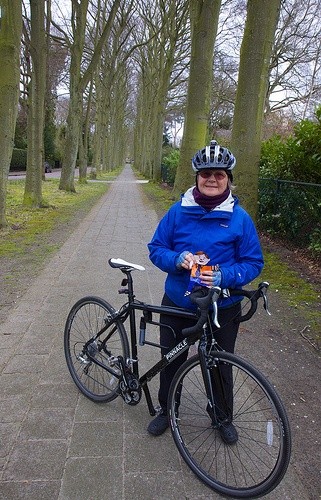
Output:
[191,145,236,172]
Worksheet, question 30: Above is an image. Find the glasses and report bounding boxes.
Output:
[197,171,226,180]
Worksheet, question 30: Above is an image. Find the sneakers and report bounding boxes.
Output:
[147,410,178,436]
[215,419,238,443]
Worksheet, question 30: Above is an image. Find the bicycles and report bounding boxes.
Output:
[63,255,292,499]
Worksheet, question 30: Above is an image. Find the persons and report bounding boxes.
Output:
[148,140,264,443]
